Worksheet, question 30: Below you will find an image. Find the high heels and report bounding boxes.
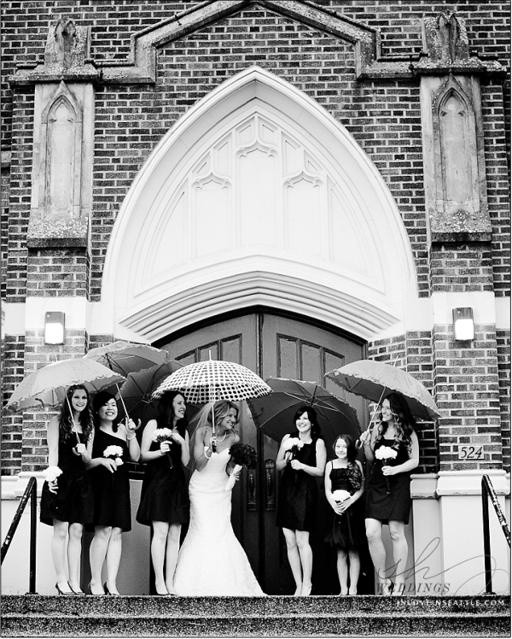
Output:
[294,579,404,596]
[55,582,175,596]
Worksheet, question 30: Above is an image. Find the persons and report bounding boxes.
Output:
[324,434,365,596]
[39,384,118,596]
[173,400,269,596]
[359,393,420,596]
[273,406,327,596]
[135,394,190,596]
[83,392,141,596]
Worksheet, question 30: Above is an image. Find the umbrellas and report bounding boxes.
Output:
[246,377,361,443]
[325,359,442,448]
[115,360,184,469]
[151,350,272,451]
[2,358,128,456]
[82,341,170,430]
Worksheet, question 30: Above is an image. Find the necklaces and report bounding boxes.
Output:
[214,431,226,443]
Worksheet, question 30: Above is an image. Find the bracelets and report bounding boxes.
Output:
[126,432,137,440]
[203,452,212,460]
